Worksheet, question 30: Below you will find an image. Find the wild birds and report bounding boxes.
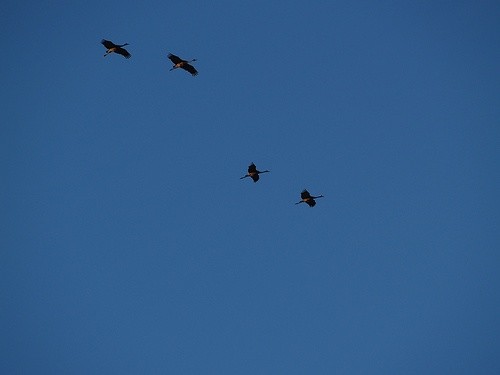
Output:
[168,53,199,77]
[240,162,270,183]
[295,189,323,208]
[100,39,132,60]
